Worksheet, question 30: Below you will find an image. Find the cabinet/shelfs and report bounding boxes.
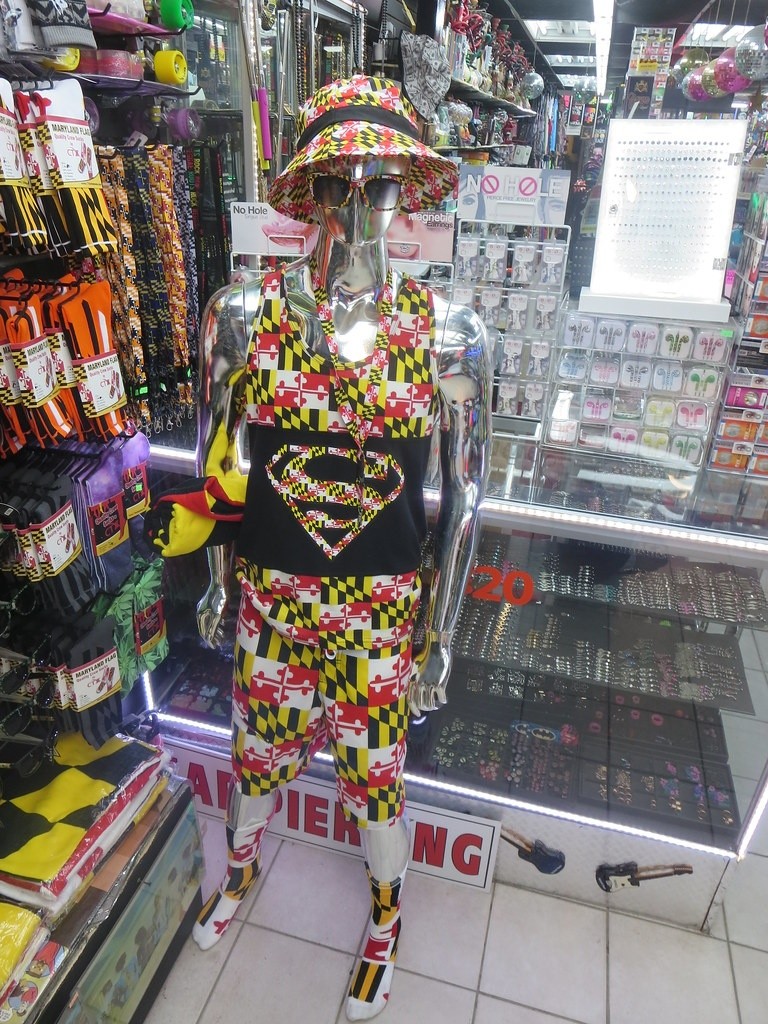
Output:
[147,532,768,841]
[705,174,768,479]
[415,0,565,150]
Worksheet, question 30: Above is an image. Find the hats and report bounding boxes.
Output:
[266,75,459,225]
[400,30,453,122]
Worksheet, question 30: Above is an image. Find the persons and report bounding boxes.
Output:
[193,75,492,1021]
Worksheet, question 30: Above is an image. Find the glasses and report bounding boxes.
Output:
[308,171,406,212]
[0,518,63,776]
